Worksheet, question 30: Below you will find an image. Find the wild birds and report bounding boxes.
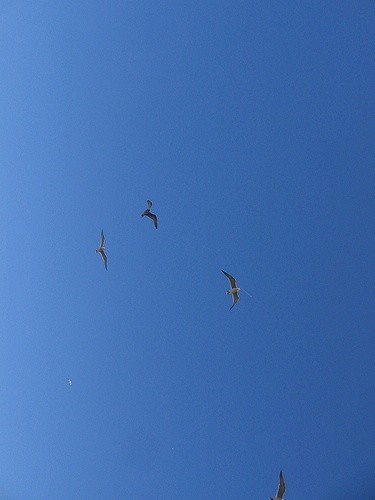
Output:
[96,228,108,271]
[268,470,286,500]
[221,270,241,311]
[141,200,157,229]
